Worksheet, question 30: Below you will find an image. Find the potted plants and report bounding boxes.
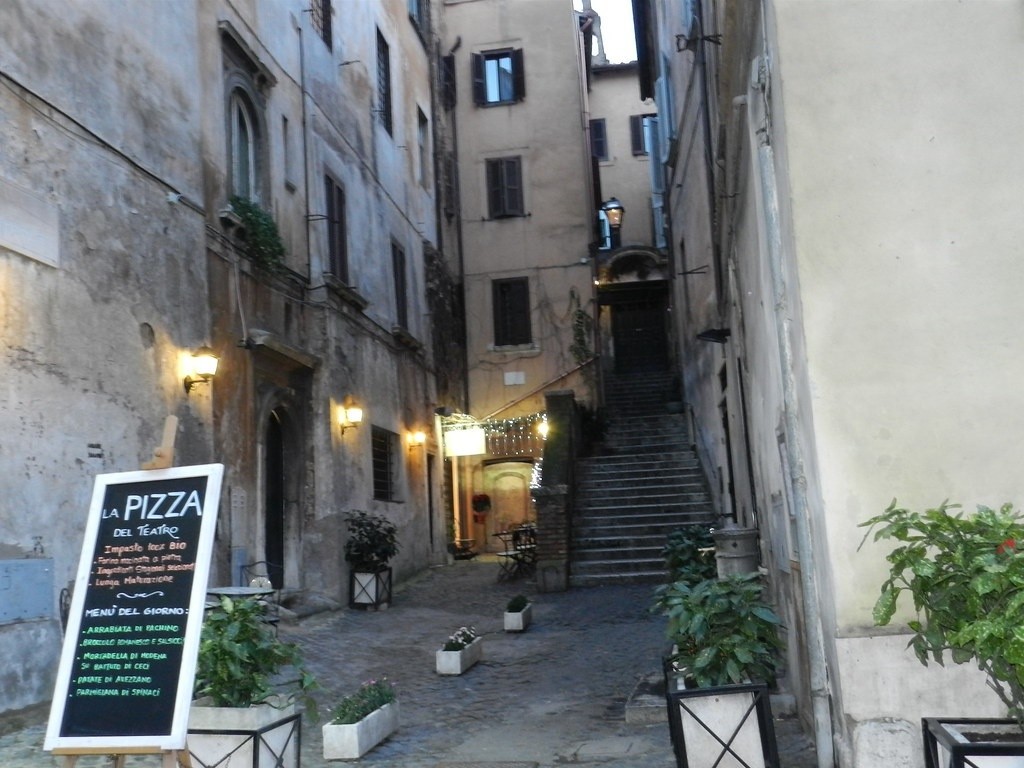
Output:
[649,577,780,768]
[857,501,1024,768]
[344,510,392,606]
[181,592,320,768]
[504,595,532,630]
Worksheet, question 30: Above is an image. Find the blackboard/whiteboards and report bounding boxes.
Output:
[42,464,225,750]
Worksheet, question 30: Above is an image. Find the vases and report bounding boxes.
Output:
[436,637,484,675]
[322,701,400,758]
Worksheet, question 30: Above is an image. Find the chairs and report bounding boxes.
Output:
[448,542,467,561]
[492,523,537,583]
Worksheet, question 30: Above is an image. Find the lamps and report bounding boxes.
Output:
[339,401,362,435]
[696,327,733,344]
[590,196,626,257]
[180,342,222,392]
[405,431,427,447]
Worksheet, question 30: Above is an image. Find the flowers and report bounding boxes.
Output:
[336,676,401,724]
[444,626,477,651]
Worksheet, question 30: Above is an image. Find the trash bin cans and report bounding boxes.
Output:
[712,524,760,581]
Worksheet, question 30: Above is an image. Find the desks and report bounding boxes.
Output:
[493,527,536,571]
[456,539,476,561]
